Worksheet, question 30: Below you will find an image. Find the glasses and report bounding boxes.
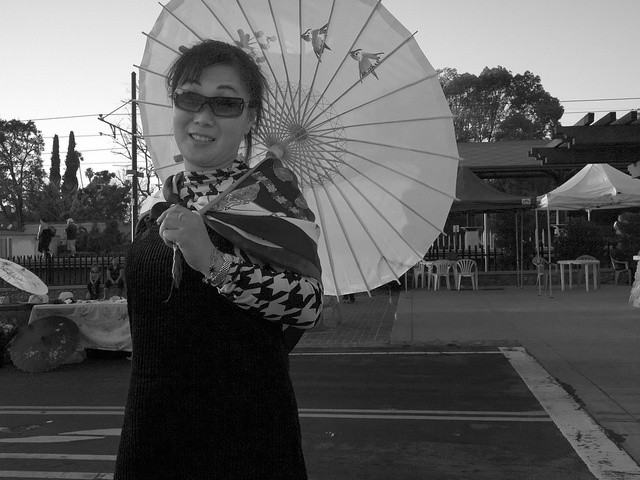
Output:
[170,90,244,118]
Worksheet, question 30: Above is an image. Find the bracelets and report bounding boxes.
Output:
[202,242,219,284]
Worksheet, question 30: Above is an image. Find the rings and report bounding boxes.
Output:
[177,212,186,221]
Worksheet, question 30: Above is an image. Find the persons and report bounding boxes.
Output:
[87,264,101,299]
[610,242,634,286]
[342,294,358,303]
[613,214,624,234]
[29,295,49,303]
[65,218,79,258]
[100,257,125,300]
[36,217,54,259]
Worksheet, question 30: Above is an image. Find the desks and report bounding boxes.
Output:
[28,298,132,359]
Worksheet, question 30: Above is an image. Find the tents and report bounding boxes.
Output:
[405,165,536,293]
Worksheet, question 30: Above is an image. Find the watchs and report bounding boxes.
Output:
[209,252,237,287]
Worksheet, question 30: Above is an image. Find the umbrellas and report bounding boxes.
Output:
[124,0,467,304]
[6,315,80,374]
[534,164,640,300]
[0,258,49,305]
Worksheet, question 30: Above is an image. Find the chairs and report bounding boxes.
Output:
[456,259,479,290]
[412,260,426,289]
[430,259,450,290]
[533,256,558,291]
[577,255,600,288]
[608,253,631,286]
